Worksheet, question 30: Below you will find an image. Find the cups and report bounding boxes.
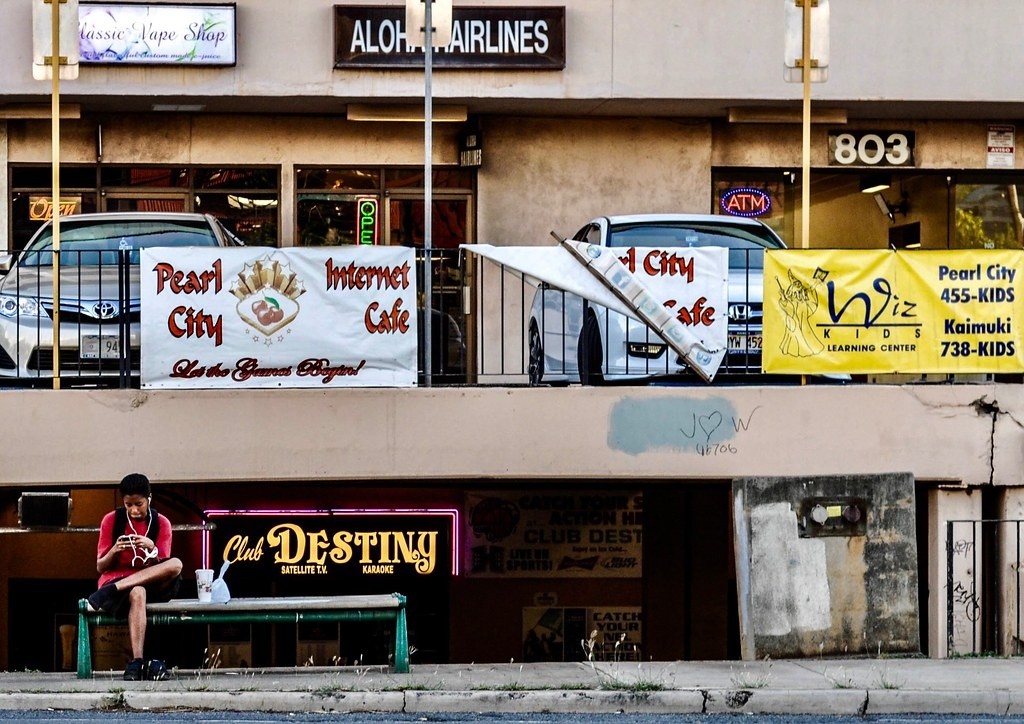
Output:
[195,569,214,602]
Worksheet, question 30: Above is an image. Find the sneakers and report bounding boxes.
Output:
[123,657,170,681]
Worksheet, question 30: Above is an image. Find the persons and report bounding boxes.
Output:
[83,471,185,682]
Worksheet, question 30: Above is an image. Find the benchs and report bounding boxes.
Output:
[76,592,413,679]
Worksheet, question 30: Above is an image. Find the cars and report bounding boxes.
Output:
[0,209,251,389]
[416,304,467,375]
[525,212,854,387]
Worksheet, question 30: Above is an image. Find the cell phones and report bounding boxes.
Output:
[121,535,133,550]
